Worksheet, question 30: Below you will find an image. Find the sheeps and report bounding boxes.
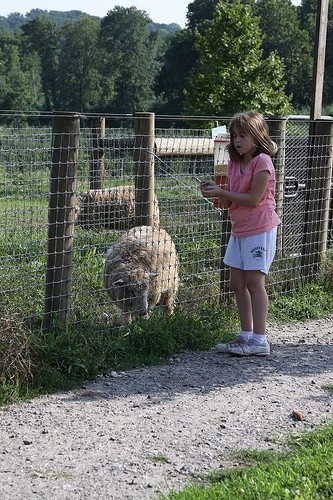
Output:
[103,226,180,328]
[75,185,160,234]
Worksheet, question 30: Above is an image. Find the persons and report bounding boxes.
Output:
[199,111,280,356]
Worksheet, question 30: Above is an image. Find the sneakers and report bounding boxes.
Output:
[228,339,270,356]
[215,335,248,353]
